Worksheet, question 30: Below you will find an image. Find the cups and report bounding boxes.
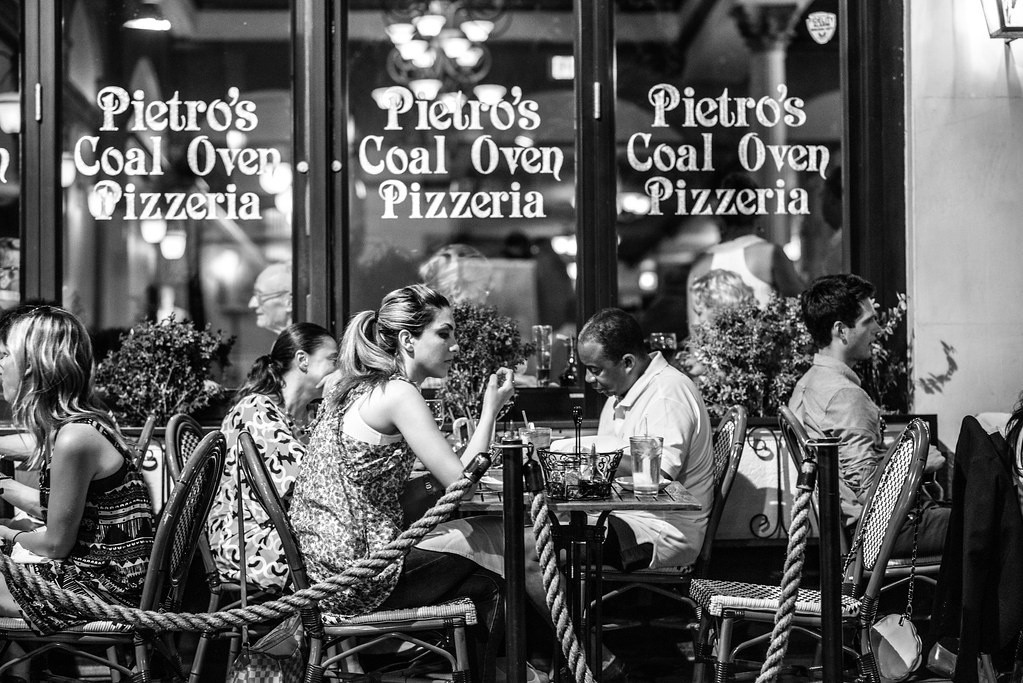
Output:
[519,427,551,483]
[630,436,663,497]
[453,422,467,451]
[425,400,443,431]
[466,420,496,446]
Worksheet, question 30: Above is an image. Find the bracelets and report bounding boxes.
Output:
[13,530,27,543]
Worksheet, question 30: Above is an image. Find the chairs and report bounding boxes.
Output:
[0,413,478,683]
[566,405,1023,683]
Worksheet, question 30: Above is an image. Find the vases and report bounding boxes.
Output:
[463,410,497,455]
[750,386,772,417]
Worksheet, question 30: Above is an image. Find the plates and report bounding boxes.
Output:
[616,476,671,492]
[480,476,503,490]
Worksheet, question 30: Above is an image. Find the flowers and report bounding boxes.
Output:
[427,303,528,418]
[678,292,909,415]
[91,318,236,426]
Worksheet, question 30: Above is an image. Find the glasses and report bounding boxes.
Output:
[253,289,292,303]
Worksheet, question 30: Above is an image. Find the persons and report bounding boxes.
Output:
[687,175,801,355]
[205,323,368,683]
[290,284,549,683]
[1005,390,1023,520]
[500,230,574,332]
[0,305,157,683]
[248,263,292,335]
[789,273,958,678]
[525,307,714,683]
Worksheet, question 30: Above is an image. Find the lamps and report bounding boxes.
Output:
[159,219,188,259]
[124,0,172,32]
[372,0,515,94]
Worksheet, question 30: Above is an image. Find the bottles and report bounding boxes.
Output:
[551,458,591,497]
[522,452,544,492]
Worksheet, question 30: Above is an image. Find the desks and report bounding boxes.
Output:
[430,484,703,683]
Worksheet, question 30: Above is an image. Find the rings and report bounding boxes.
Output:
[512,381,515,384]
